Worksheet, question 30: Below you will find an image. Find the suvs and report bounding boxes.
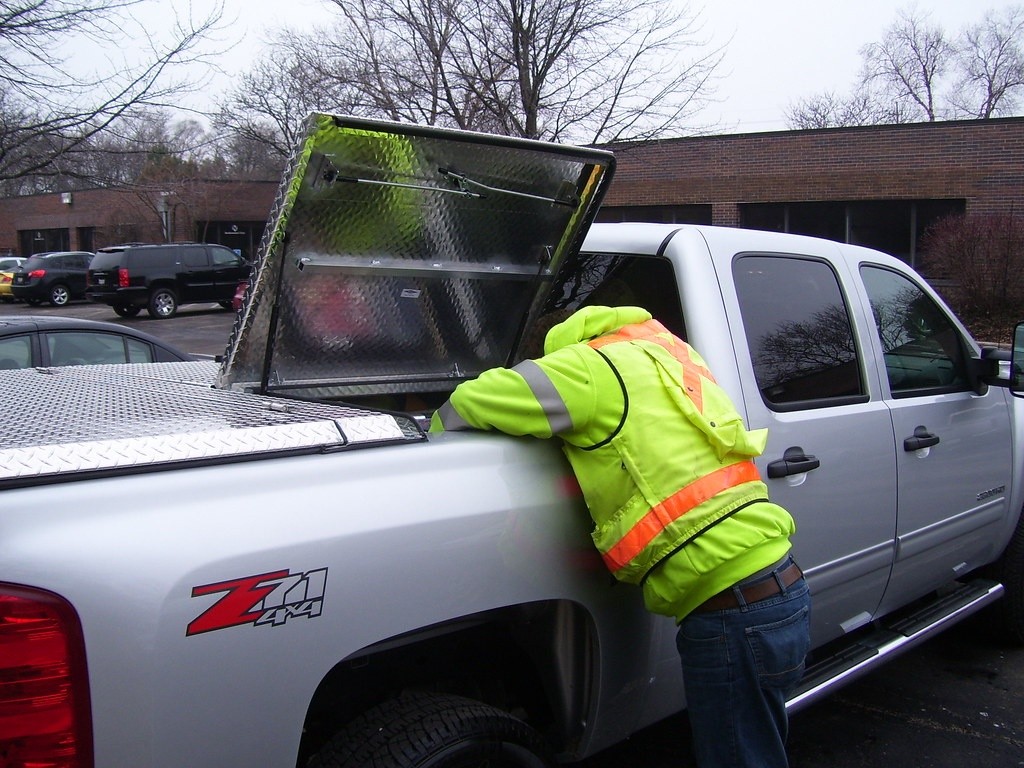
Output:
[10,251,96,308]
[84,241,255,319]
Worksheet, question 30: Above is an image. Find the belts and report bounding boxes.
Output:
[694,561,802,614]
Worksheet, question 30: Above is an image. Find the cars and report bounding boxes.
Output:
[0,315,217,370]
[0,257,28,302]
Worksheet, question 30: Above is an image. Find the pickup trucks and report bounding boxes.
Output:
[0,112,1024,768]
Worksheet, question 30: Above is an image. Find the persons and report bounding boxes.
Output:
[429,305,810,768]
